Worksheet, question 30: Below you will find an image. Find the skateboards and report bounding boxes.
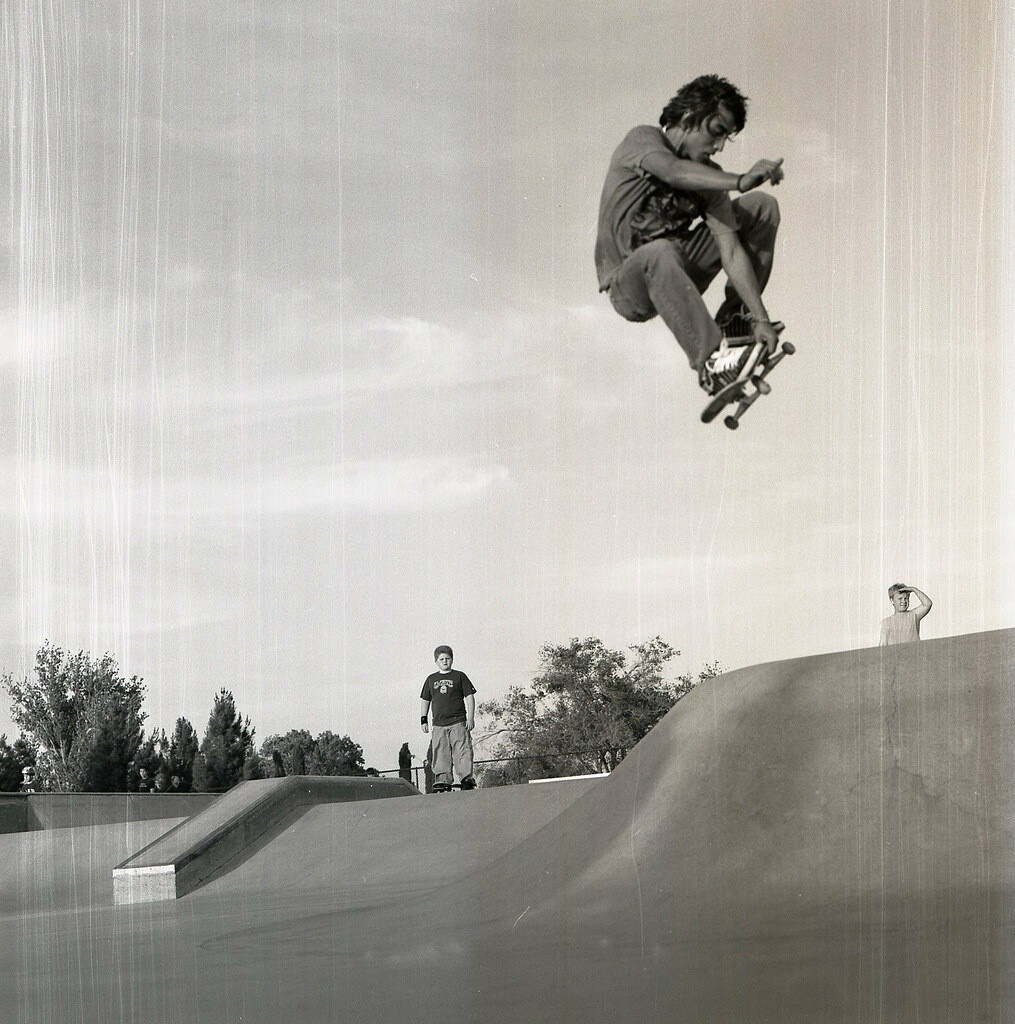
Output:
[700,327,796,431]
[432,781,476,792]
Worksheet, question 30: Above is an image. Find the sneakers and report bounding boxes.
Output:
[719,313,784,345]
[697,338,763,398]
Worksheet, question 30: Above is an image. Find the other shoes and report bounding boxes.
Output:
[462,778,476,787]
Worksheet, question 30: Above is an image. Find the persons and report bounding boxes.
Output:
[419,645,478,793]
[399,742,415,783]
[593,72,785,399]
[879,583,933,645]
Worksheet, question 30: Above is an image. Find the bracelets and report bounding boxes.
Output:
[737,173,744,194]
[421,715,428,725]
[751,318,769,327]
